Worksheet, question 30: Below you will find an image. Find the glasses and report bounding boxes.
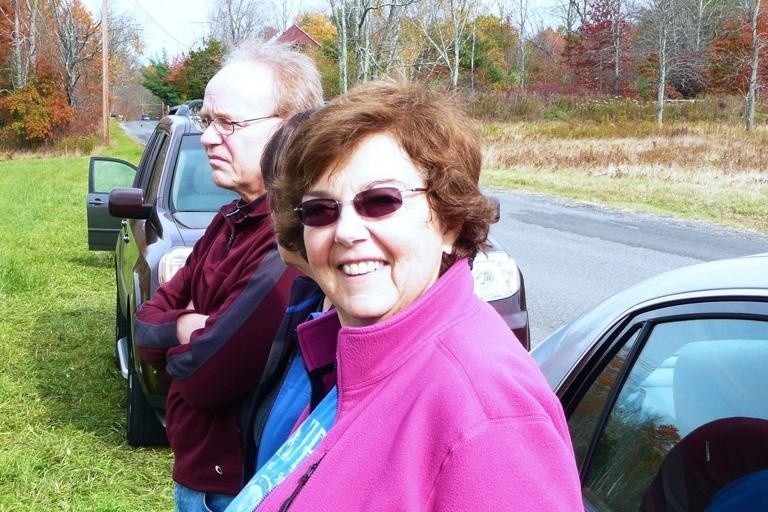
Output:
[292,187,430,227]
[190,114,281,135]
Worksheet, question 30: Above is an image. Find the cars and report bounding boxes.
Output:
[528,252,768,512]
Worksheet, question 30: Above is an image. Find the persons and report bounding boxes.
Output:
[135,37,325,512]
[226,79,585,512]
[239,106,334,491]
